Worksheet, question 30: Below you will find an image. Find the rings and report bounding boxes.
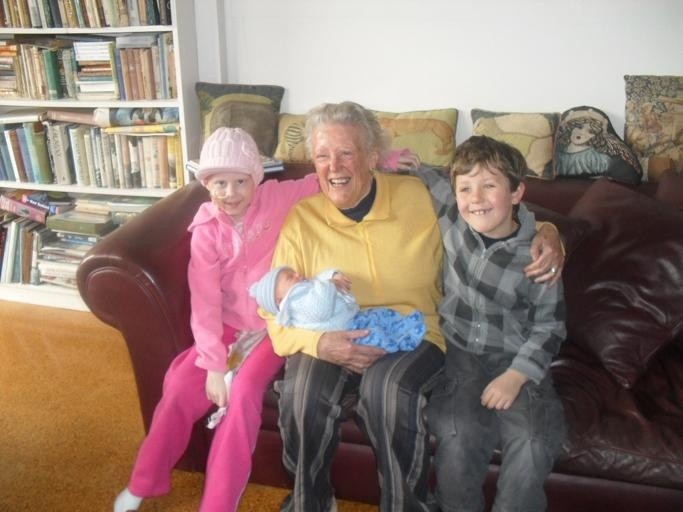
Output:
[550,268,557,273]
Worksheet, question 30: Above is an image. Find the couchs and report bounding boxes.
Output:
[69,146,683,512]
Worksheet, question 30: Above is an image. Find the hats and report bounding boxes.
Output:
[249,265,295,316]
[194,125,264,188]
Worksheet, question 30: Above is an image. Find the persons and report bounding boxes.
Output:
[371,135,565,512]
[257,101,565,512]
[557,110,612,176]
[112,127,423,511]
[247,266,428,353]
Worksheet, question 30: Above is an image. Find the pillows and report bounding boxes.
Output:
[470,106,559,183]
[521,201,590,273]
[191,75,285,155]
[619,73,682,162]
[358,106,460,170]
[551,103,640,186]
[275,110,311,163]
[566,174,683,394]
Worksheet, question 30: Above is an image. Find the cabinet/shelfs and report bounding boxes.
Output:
[0,1,204,311]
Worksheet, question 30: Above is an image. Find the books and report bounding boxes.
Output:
[1,32,177,101]
[1,188,163,288]
[1,0,172,28]
[0,106,181,191]
